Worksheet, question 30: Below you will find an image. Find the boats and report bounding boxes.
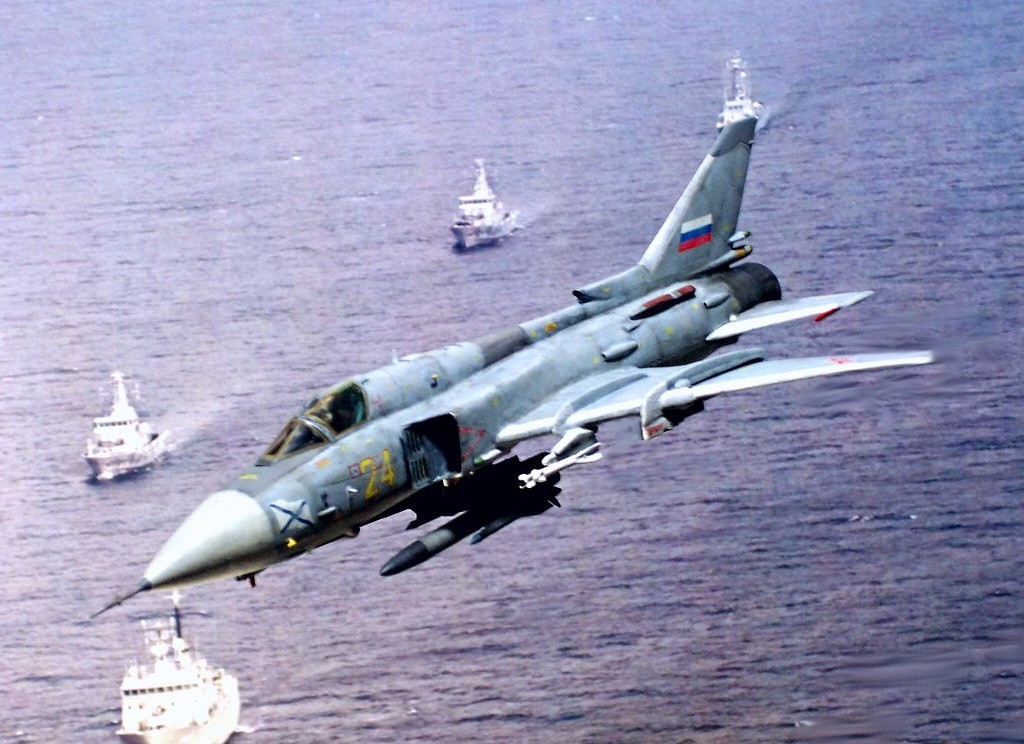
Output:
[114,588,240,744]
[717,53,763,133]
[450,158,516,252]
[85,370,167,475]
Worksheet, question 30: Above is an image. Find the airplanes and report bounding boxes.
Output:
[84,114,932,621]
